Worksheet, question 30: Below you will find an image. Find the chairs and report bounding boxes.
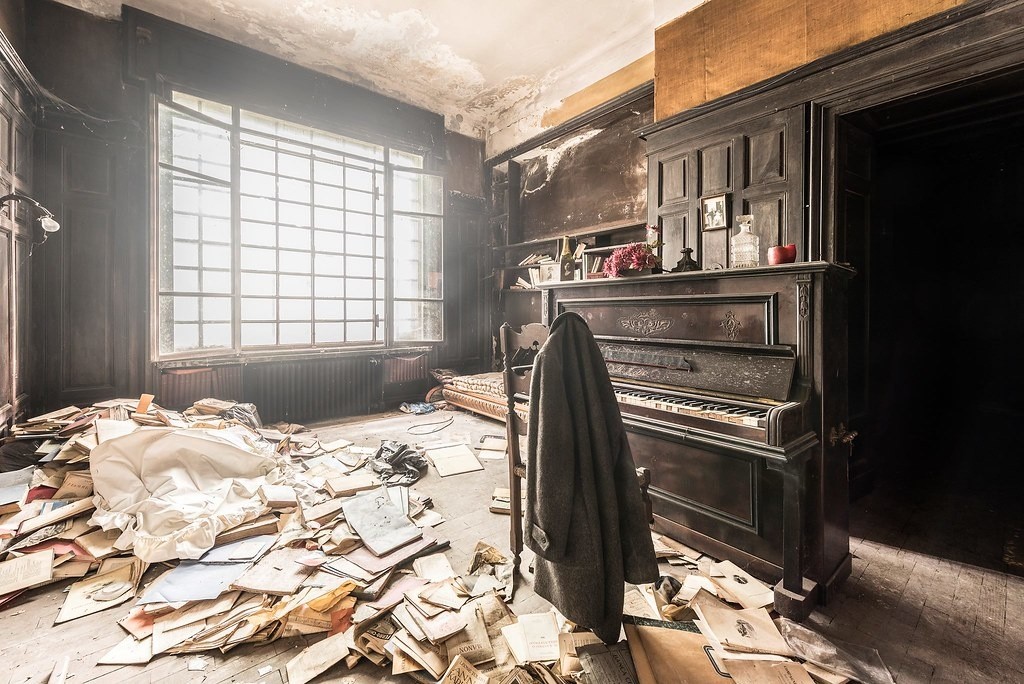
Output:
[499,321,652,575]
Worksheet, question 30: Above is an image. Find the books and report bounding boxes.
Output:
[506,242,610,291]
[0,394,891,683]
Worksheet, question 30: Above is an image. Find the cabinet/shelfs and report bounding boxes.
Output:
[493,222,647,295]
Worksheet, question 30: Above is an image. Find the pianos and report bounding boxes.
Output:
[501,262,866,615]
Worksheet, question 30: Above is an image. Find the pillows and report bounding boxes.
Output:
[429,368,461,385]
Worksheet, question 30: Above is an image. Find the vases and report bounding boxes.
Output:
[621,268,662,277]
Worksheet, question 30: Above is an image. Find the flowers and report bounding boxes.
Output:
[603,224,666,279]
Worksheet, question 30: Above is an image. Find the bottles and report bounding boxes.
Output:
[731,215,759,267]
[560,235,574,280]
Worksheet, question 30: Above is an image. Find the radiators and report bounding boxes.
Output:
[246,358,381,422]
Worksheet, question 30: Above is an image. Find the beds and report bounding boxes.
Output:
[442,371,530,426]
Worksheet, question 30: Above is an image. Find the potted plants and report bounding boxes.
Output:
[784,242,796,263]
[767,239,785,265]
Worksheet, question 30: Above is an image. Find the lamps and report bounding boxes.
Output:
[0,194,61,233]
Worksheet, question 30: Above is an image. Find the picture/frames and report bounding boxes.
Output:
[699,193,729,232]
[539,262,560,282]
